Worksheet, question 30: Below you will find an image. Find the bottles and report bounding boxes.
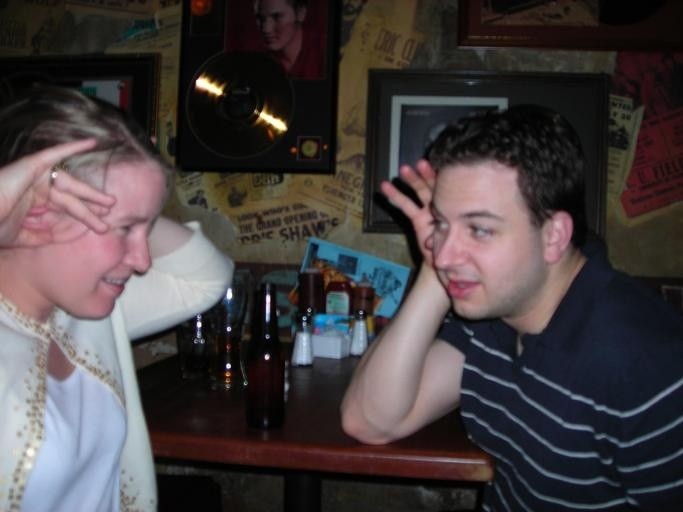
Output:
[169,275,292,432]
[288,311,314,367]
[349,308,370,359]
[325,273,351,318]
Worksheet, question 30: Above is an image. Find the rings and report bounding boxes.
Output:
[49,168,55,187]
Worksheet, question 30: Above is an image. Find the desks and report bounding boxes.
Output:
[137,352,493,509]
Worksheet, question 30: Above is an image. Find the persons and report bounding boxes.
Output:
[0,86,236,512]
[251,1,319,80]
[336,104,683,512]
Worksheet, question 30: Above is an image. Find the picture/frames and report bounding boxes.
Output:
[361,67,613,234]
[2,51,163,173]
[175,3,342,176]
[455,3,682,54]
